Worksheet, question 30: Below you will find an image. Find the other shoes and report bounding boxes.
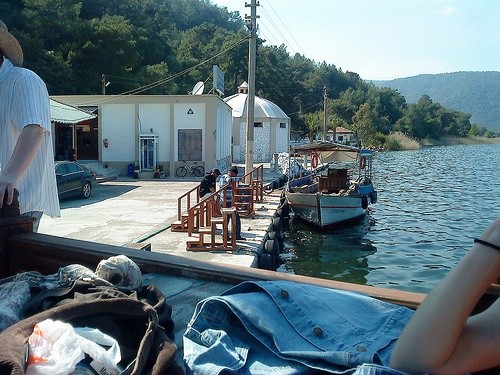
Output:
[226,237,231,241]
[236,237,246,240]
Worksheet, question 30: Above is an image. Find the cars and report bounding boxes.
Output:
[53,161,96,199]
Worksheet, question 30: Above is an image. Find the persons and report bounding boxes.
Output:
[219,166,246,240]
[199,168,222,216]
[391,215,500,375]
[0,20,61,233]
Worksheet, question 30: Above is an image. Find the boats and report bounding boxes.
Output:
[285,140,378,229]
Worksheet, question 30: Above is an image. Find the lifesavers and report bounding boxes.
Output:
[258,192,290,271]
[311,154,318,168]
[361,195,368,209]
[371,191,377,204]
[359,156,366,169]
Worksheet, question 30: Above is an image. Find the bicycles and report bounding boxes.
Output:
[176,160,205,177]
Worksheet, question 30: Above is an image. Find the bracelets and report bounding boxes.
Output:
[474,238,500,251]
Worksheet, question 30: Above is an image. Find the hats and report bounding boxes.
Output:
[0,20,23,67]
[214,169,220,175]
